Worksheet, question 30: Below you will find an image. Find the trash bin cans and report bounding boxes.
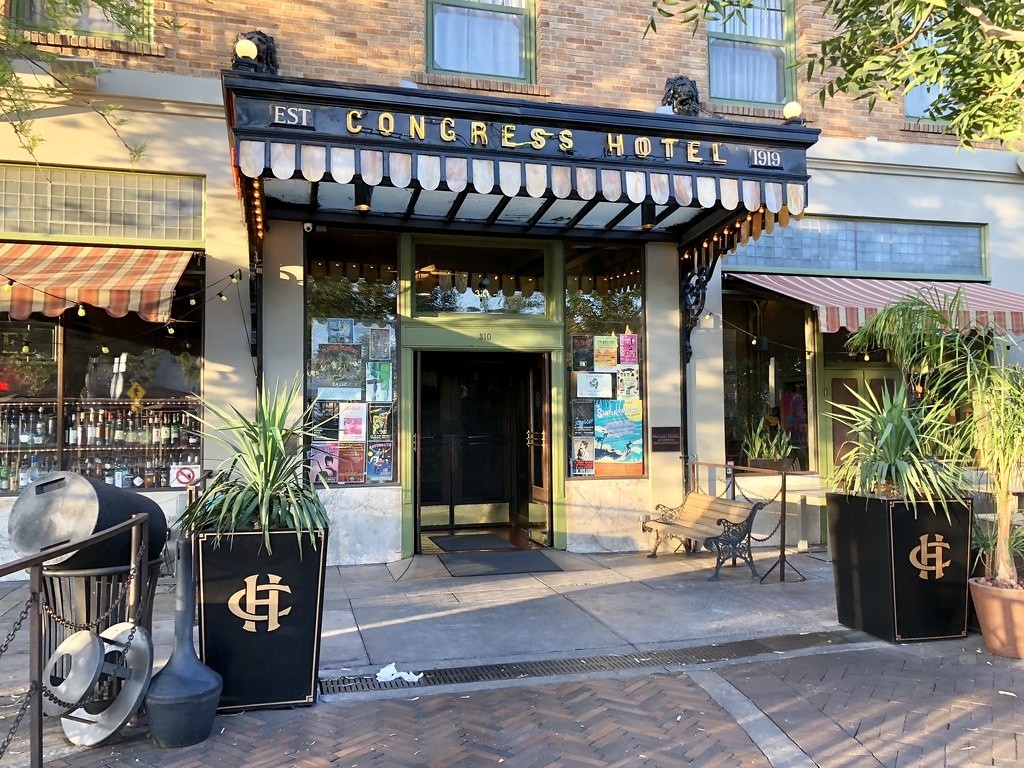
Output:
[26,557,165,716]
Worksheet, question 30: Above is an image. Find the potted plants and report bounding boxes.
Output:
[169,370,347,716]
[843,285,1024,660]
[820,376,974,646]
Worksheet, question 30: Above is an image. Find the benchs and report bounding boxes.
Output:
[641,460,806,583]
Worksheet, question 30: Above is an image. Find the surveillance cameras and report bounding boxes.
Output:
[304,223,313,233]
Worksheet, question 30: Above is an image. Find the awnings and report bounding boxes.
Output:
[728,274,1024,337]
[0,244,194,324]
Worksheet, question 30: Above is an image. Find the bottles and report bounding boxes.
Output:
[0,408,198,447]
[0,453,199,493]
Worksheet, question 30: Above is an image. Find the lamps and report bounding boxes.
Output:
[783,102,802,119]
[235,39,258,60]
